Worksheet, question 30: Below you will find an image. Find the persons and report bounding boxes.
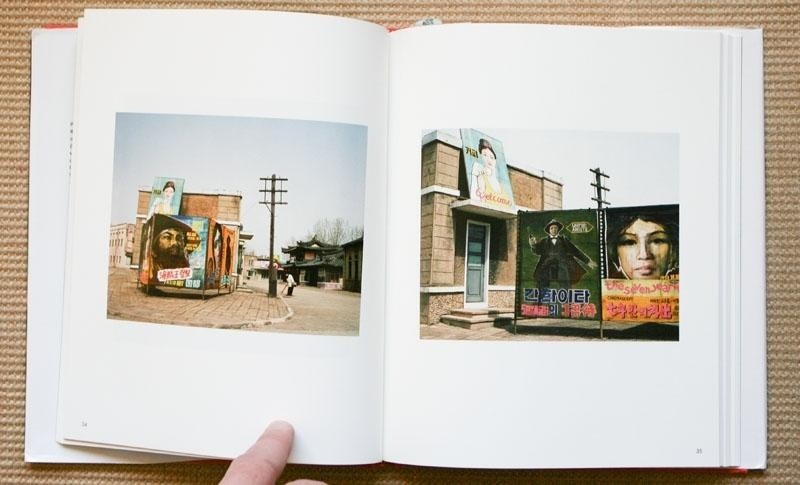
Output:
[285,273,296,296]
[607,215,679,280]
[207,257,213,277]
[218,420,327,485]
[147,181,175,220]
[469,139,510,209]
[212,223,222,277]
[528,219,599,306]
[152,214,190,269]
[225,235,231,288]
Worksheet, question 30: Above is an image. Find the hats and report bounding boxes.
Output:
[544,219,564,234]
[153,214,201,256]
